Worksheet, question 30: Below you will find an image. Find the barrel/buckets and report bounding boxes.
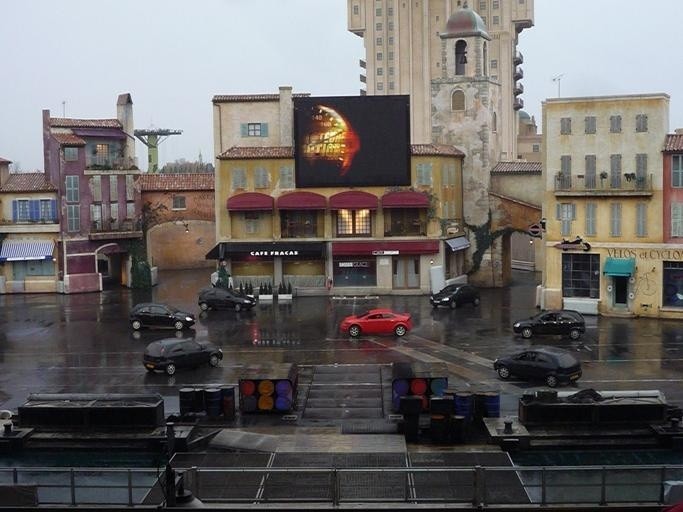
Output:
[391,363,500,438]
[239,381,292,412]
[179,385,235,419]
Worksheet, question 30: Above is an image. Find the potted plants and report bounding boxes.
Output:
[239,281,293,300]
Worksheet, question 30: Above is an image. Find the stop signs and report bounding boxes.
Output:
[529,224,541,236]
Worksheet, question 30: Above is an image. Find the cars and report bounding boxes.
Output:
[339,308,413,338]
[198,288,256,311]
[494,345,582,387]
[143,337,224,375]
[128,303,196,331]
[513,310,586,340]
[430,284,481,309]
